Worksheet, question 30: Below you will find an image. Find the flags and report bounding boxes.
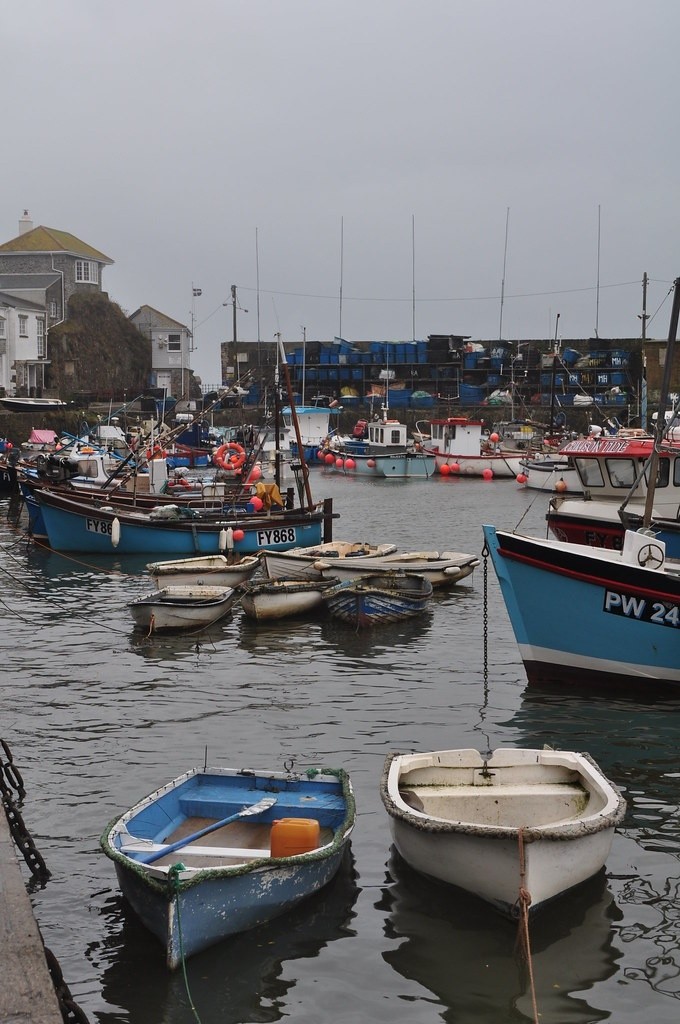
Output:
[193,289,202,296]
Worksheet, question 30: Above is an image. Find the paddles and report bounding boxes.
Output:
[137,797,278,863]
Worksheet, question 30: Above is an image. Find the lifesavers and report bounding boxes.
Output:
[167,479,191,492]
[216,442,246,470]
[147,445,166,461]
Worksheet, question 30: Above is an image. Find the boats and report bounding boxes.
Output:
[378,740,626,925]
[96,740,360,973]
[0,302,677,698]
